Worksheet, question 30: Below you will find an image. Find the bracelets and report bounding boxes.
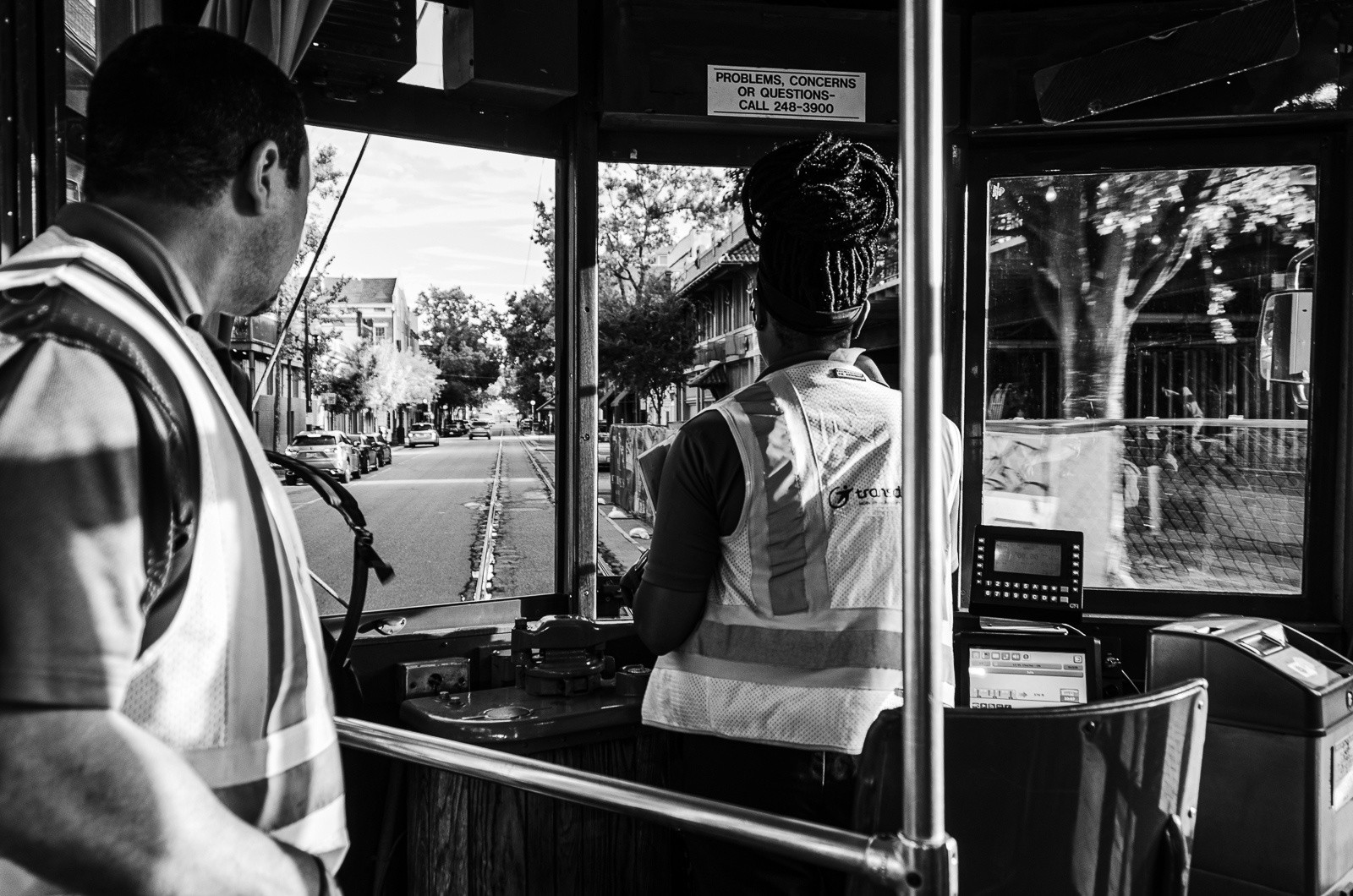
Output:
[314,855,331,896]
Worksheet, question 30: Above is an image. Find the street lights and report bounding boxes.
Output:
[530,400,535,436]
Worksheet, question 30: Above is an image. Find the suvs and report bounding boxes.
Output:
[468,421,492,440]
[284,429,362,484]
[408,422,439,447]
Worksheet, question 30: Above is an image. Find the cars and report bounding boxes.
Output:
[443,418,479,438]
[364,432,392,467]
[520,420,530,430]
[346,434,379,474]
[598,432,610,465]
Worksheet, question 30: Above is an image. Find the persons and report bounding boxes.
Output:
[0,23,350,896]
[632,132,967,695]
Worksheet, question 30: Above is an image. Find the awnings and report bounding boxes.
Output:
[687,362,730,388]
[610,387,634,407]
[597,390,615,413]
